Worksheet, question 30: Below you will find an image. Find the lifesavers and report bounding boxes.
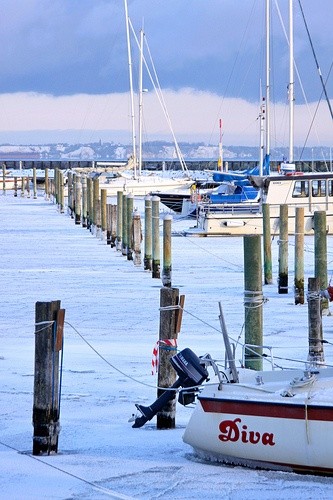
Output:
[190,192,201,203]
[286,172,302,176]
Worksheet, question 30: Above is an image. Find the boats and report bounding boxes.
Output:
[191,168,333,235]
[126,300,333,476]
